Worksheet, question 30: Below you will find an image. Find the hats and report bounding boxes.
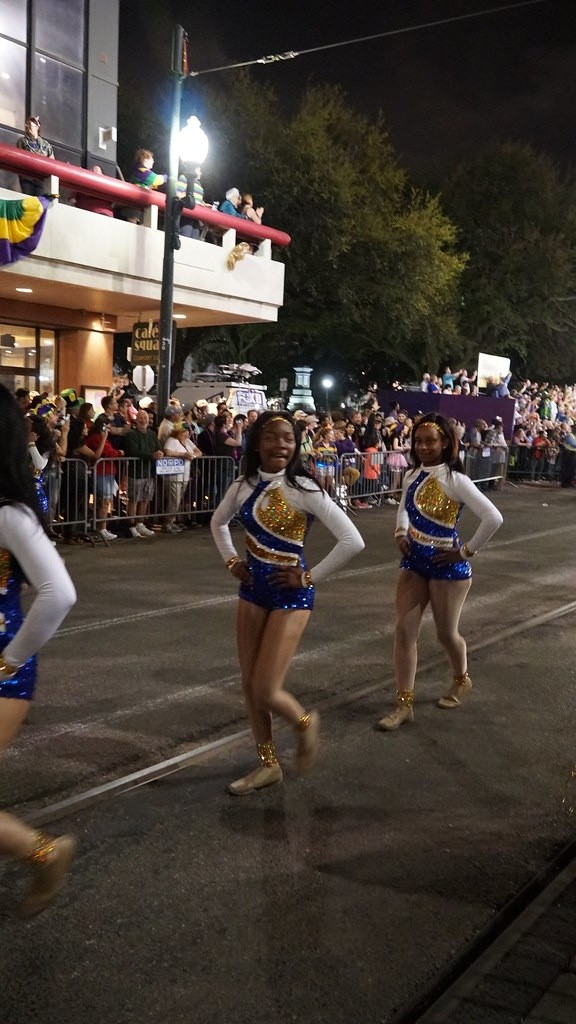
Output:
[174,422,189,431]
[37,404,53,417]
[306,414,320,424]
[384,417,398,425]
[295,411,307,417]
[164,406,180,415]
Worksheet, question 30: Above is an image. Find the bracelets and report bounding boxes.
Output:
[304,570,314,587]
[463,543,477,557]
[395,527,406,532]
[0,655,17,674]
[225,557,240,568]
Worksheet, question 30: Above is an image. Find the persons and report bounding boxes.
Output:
[69,164,114,218]
[210,411,365,796]
[14,367,576,546]
[119,148,167,224]
[0,383,78,917]
[379,412,504,731]
[14,117,55,196]
[221,186,264,224]
[177,167,212,239]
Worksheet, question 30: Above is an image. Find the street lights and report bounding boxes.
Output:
[321,378,333,404]
[156,22,210,432]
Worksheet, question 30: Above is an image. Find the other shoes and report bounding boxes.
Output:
[378,704,415,729]
[298,712,317,769]
[351,497,400,510]
[21,832,78,918]
[438,677,474,709]
[229,762,283,795]
[51,520,203,546]
